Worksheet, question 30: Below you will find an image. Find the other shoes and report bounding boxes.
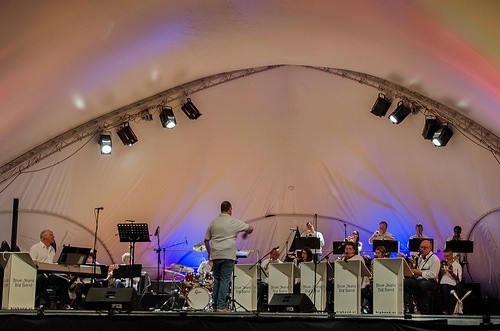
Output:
[405,305,415,313]
[213,308,215,312]
[216,307,234,312]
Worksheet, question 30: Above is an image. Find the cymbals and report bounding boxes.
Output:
[169,263,194,272]
[192,242,207,253]
[163,269,185,278]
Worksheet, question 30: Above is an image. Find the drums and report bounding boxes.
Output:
[179,281,195,300]
[184,271,203,286]
[201,271,214,287]
[187,286,212,311]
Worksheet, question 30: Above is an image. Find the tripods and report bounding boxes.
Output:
[223,251,251,312]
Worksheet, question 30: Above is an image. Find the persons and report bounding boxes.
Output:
[103,252,139,291]
[204,200,254,313]
[444,226,465,264]
[405,240,440,313]
[257,249,283,312]
[300,222,325,261]
[198,258,214,286]
[336,242,370,300]
[432,248,463,314]
[68,249,100,300]
[344,230,363,256]
[369,221,395,258]
[369,246,386,281]
[30,229,75,310]
[407,224,430,258]
[286,247,314,312]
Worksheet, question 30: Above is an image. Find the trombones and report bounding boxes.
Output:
[440,254,461,274]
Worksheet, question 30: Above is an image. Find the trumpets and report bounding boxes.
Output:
[374,229,384,240]
[306,230,314,237]
[343,235,358,242]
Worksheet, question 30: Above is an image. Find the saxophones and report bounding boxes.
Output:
[408,252,423,279]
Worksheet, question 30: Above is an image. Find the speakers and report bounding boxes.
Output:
[269,293,316,311]
[84,288,143,310]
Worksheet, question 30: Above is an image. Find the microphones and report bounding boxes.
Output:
[155,226,159,235]
[95,207,104,209]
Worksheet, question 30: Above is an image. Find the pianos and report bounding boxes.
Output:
[33,245,109,310]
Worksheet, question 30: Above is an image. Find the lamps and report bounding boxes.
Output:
[422,115,440,140]
[430,121,454,147]
[159,106,177,128]
[116,121,139,146]
[370,93,392,118]
[98,130,113,155]
[388,101,412,125]
[180,97,202,120]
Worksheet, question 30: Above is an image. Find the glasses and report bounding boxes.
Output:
[419,246,428,250]
